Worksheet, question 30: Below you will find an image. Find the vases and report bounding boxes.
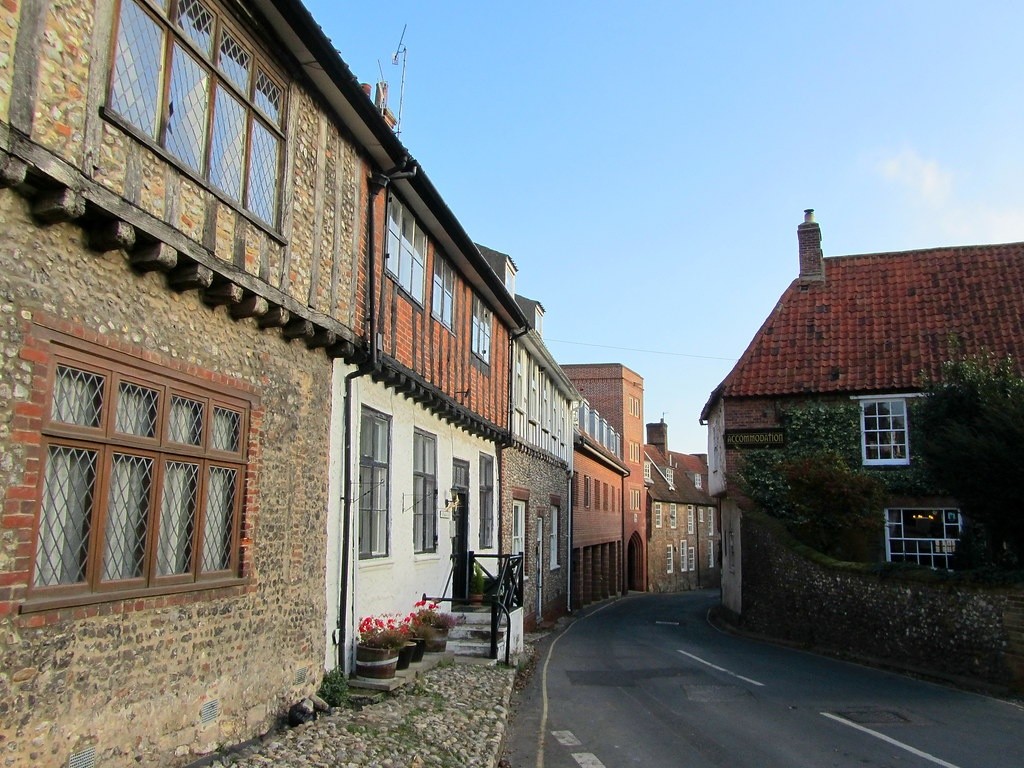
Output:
[356,643,399,679]
[397,641,417,670]
[426,628,449,652]
[410,638,428,663]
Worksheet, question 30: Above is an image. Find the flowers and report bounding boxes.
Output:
[360,600,459,650]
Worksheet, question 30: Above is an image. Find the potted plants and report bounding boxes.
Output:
[468,562,484,606]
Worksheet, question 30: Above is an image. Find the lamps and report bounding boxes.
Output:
[445,483,459,511]
